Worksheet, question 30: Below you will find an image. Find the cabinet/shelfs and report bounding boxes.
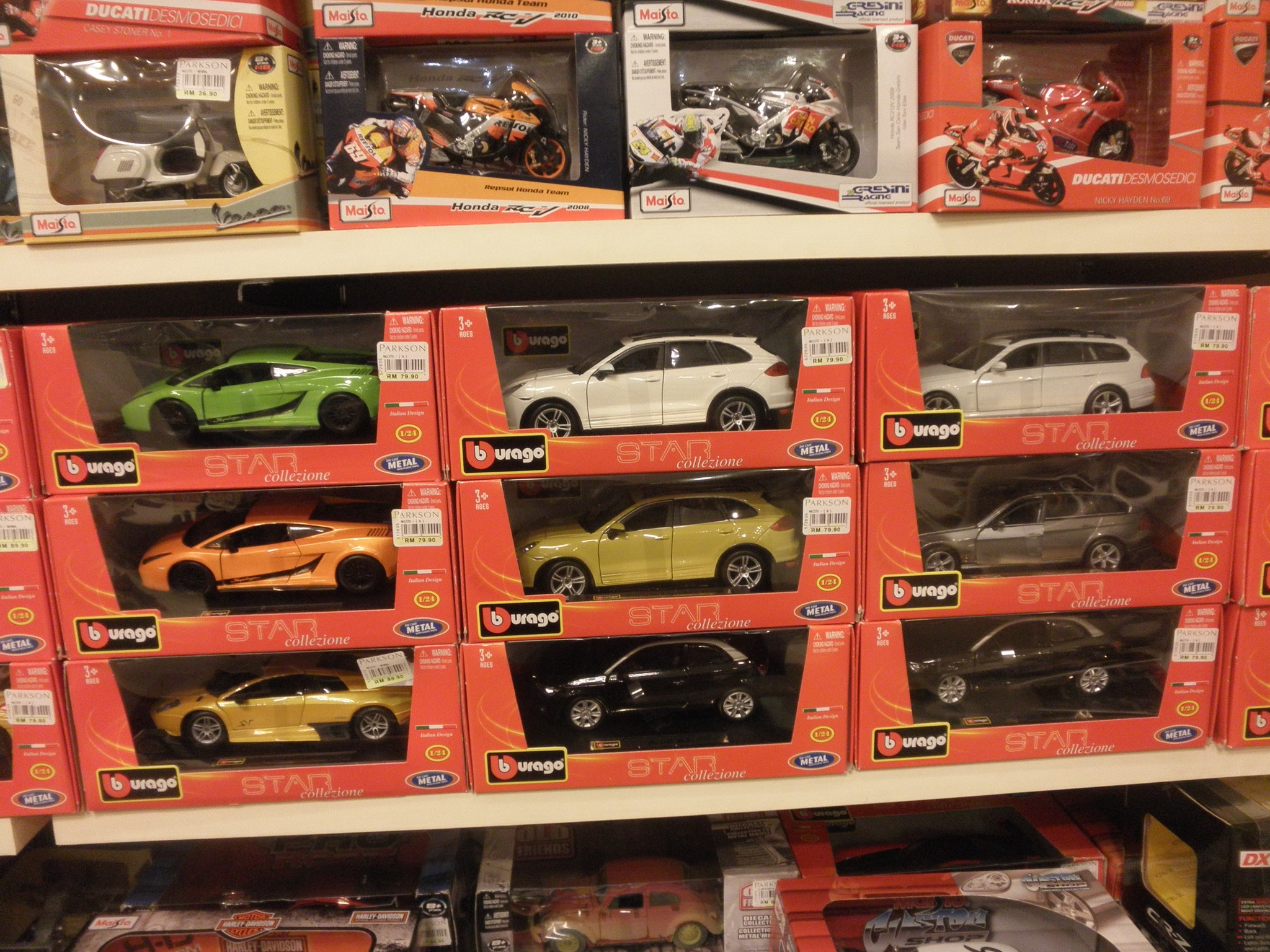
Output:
[0,206,1270,855]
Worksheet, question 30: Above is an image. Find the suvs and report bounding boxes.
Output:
[1073,371,1076,374]
[921,487,1159,586]
[506,330,793,444]
[519,484,806,600]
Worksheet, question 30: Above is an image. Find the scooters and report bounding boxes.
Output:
[91,102,253,207]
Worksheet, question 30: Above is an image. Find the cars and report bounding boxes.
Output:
[148,665,414,763]
[914,330,1158,419]
[116,346,385,448]
[536,858,722,952]
[136,494,406,604]
[908,616,1124,715]
[529,638,773,733]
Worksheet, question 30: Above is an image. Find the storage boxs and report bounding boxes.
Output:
[0,0,1270,952]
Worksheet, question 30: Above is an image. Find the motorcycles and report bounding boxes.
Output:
[986,59,1135,160]
[381,68,568,180]
[628,102,729,185]
[322,120,398,197]
[1225,127,1270,195]
[683,62,861,176]
[942,99,1070,205]
